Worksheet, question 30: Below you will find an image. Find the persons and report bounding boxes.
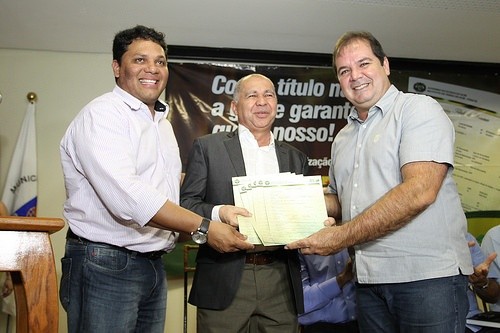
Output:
[59,25,254,333]
[285,31,474,333]
[299,247,360,333]
[179,73,310,333]
[465,225,500,333]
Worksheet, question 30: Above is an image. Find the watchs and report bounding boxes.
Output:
[191,218,210,244]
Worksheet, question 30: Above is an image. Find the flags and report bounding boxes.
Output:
[1,103,38,218]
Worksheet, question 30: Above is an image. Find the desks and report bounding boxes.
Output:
[1,216,65,333]
